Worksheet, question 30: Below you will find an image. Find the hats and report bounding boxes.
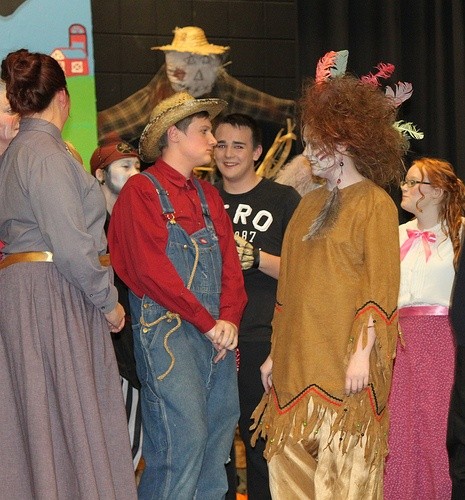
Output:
[90,131,142,177]
[138,92,228,163]
[150,26,230,56]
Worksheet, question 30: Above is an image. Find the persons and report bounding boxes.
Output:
[0,48,139,500]
[86,139,146,486]
[382,158,464,500]
[249,75,405,500]
[210,114,303,500]
[107,90,248,500]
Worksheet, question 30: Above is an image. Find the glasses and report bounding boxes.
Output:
[399,180,431,188]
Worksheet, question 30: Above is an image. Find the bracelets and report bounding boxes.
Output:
[251,248,260,272]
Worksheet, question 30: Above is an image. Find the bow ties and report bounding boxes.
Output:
[399,229,436,263]
